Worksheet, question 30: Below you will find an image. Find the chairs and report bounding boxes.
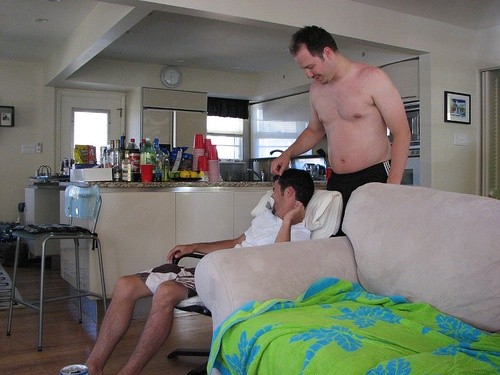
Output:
[168,188,342,374]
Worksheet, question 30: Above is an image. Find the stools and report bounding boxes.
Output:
[6,182,108,352]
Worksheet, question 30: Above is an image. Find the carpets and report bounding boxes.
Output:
[0,264,28,311]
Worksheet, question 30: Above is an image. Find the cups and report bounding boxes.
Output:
[139,164,154,181]
[325,167,331,181]
[192,134,221,181]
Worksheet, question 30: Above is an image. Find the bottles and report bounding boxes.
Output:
[99,136,193,182]
[61,157,75,176]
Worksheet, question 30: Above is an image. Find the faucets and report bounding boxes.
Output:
[247,168,265,182]
[270,149,284,155]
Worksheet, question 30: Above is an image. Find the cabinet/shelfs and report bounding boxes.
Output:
[250,59,420,159]
[141,87,207,154]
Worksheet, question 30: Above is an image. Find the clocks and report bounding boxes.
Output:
[160,65,182,88]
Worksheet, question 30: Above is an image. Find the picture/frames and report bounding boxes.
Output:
[0,106,14,126]
[444,91,471,124]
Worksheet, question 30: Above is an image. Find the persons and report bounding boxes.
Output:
[83,167,315,375]
[272,26,411,237]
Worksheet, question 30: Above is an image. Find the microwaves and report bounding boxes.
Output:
[385,109,420,158]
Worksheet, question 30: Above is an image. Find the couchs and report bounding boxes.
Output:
[194,181,500,374]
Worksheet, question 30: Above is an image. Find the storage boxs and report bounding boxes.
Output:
[69,168,112,182]
